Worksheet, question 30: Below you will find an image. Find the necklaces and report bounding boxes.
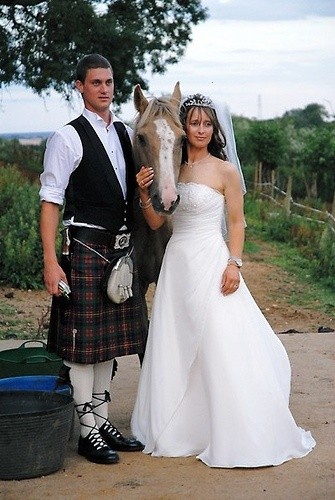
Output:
[184,152,211,168]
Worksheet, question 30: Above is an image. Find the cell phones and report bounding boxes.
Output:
[58,280,71,298]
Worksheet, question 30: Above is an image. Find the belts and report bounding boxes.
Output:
[66,226,138,251]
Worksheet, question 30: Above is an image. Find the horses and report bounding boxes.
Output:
[126,79,187,294]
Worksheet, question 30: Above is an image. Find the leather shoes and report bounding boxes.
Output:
[77,433,119,463]
[99,423,143,452]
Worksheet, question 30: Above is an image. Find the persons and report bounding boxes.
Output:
[131,93,318,468]
[39,54,148,465]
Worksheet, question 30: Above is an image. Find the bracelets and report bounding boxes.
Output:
[139,197,151,204]
[138,200,151,209]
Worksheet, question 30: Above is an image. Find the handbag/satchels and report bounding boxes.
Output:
[101,254,135,305]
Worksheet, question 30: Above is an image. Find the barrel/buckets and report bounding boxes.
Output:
[0,340,74,480]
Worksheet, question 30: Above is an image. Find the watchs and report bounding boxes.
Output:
[227,257,243,268]
